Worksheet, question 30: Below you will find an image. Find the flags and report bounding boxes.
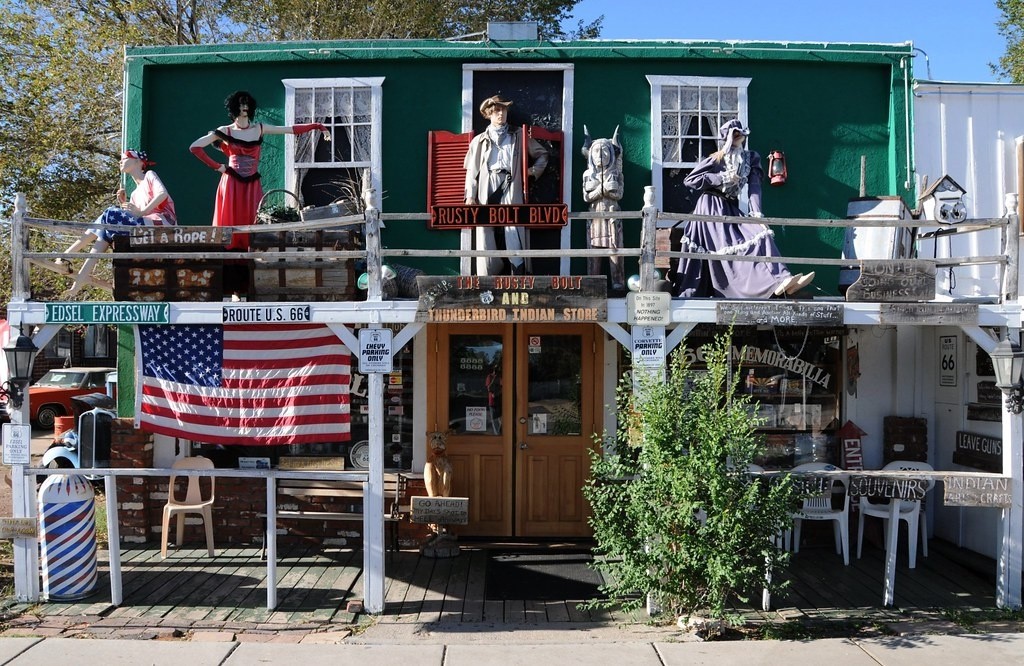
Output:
[131,323,353,444]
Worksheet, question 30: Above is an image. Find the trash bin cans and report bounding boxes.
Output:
[70,392,116,428]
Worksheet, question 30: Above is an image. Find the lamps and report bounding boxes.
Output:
[767,149,789,188]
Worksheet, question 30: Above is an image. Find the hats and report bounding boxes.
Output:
[720,119,749,142]
[480,95,513,116]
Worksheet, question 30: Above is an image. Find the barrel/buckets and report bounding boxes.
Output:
[54,416,75,437]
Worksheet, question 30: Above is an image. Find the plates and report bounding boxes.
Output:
[350,441,369,467]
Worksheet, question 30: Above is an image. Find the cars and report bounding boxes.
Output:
[26,367,117,432]
[527,400,581,437]
[449,418,499,435]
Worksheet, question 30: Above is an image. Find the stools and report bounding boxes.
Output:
[783,461,851,566]
[160,456,217,562]
[857,459,936,570]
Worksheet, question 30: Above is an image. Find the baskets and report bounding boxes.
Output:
[254,190,302,224]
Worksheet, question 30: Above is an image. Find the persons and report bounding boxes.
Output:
[582,138,625,290]
[678,117,816,299]
[486,363,503,434]
[462,95,549,277]
[53,149,175,296]
[189,89,328,296]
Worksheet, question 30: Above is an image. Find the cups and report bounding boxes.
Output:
[288,443,300,455]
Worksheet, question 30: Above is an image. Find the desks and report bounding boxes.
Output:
[113,234,224,302]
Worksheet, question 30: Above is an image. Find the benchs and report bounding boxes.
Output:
[258,472,407,565]
